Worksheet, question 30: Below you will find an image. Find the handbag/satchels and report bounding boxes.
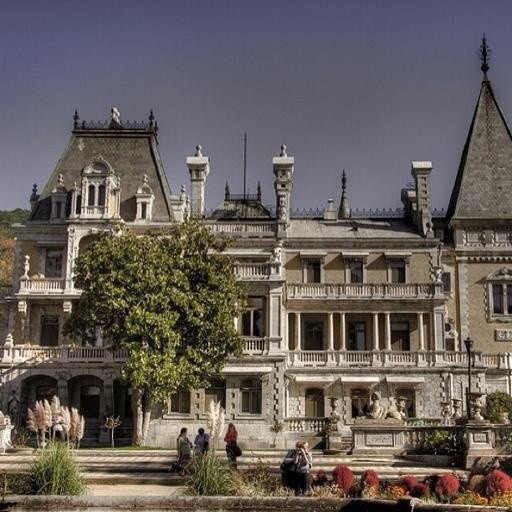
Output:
[228,446,241,456]
[173,463,181,470]
[285,465,306,490]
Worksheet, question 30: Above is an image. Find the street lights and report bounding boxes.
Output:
[464,337,474,418]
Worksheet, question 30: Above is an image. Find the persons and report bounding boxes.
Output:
[194,428,210,457]
[280,440,313,496]
[224,423,238,469]
[177,427,194,476]
